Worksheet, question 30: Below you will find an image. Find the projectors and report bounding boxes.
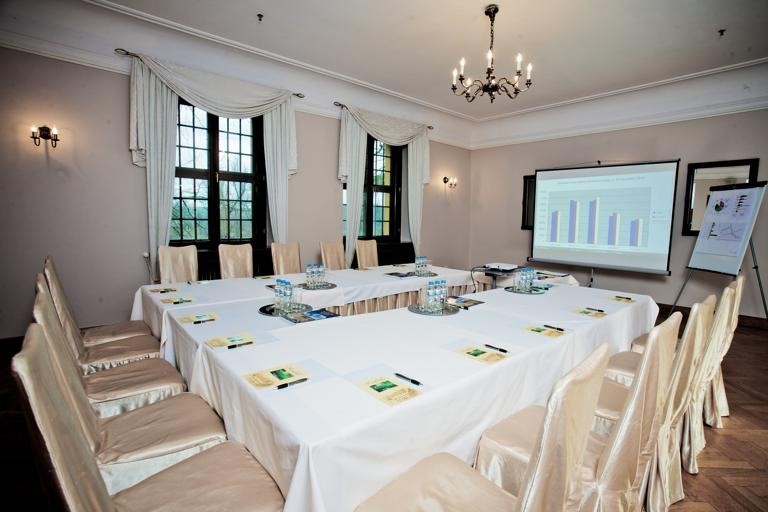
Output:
[483,263,518,277]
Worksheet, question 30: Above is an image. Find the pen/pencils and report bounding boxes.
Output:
[536,286,549,290]
[485,344,507,354]
[396,372,423,386]
[173,299,191,304]
[616,296,631,300]
[394,264,406,267]
[160,290,176,293]
[274,378,308,390]
[227,342,253,349]
[458,306,468,310]
[587,308,604,313]
[194,318,215,324]
[188,282,191,285]
[544,324,564,332]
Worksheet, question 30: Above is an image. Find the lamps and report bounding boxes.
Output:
[451,4,532,104]
[30,124,60,148]
[443,177,457,188]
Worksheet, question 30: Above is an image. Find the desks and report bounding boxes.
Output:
[131,257,661,512]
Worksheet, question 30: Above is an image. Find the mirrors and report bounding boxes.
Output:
[681,159,760,236]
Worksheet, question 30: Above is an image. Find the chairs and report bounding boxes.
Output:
[30,292,229,500]
[42,253,163,371]
[37,273,182,412]
[353,240,380,269]
[318,238,349,272]
[605,271,746,478]
[472,309,683,511]
[218,241,253,280]
[13,322,286,512]
[270,240,302,275]
[158,243,200,284]
[593,293,720,512]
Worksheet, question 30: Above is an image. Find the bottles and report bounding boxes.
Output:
[514,267,536,293]
[414,256,449,313]
[272,263,326,313]
